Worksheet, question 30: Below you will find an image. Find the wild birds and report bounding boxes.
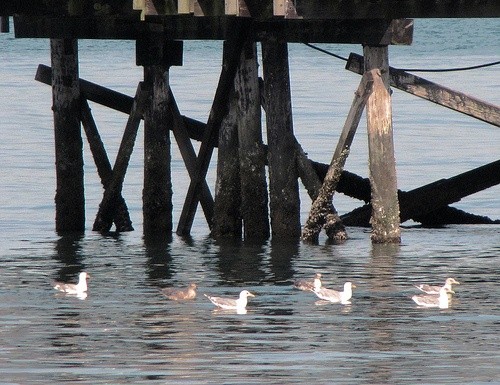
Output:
[402,285,456,309]
[156,283,200,301]
[291,273,322,291]
[308,282,356,303]
[411,277,460,295]
[47,271,91,294]
[204,289,256,310]
